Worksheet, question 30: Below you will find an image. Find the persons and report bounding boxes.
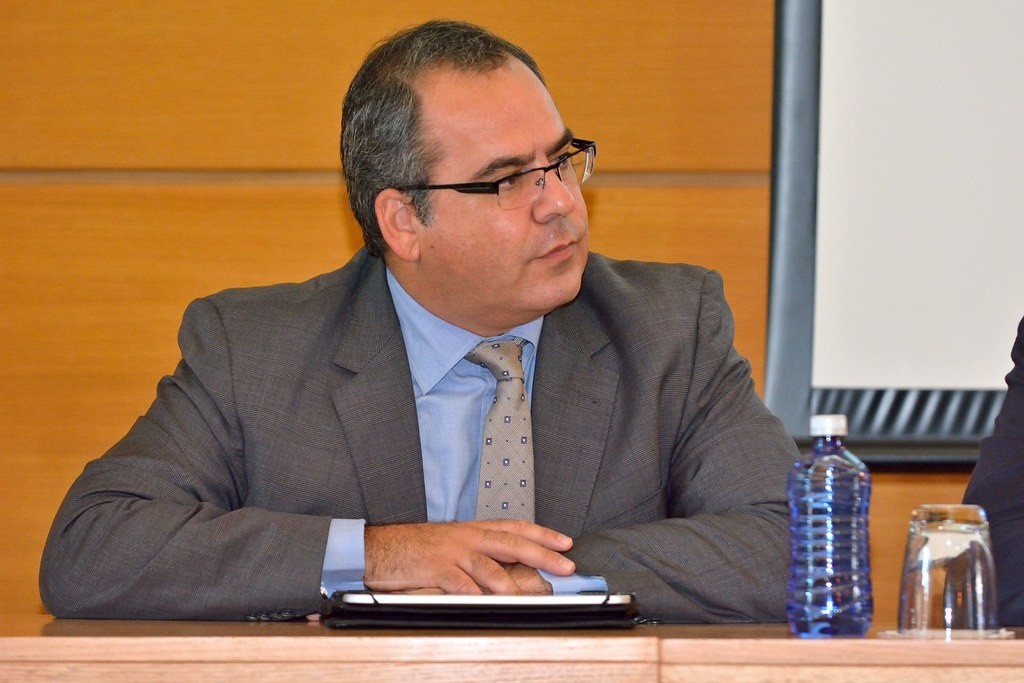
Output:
[957,315,1023,626]
[38,18,807,622]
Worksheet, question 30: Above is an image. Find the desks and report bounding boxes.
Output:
[0,610,1024,683]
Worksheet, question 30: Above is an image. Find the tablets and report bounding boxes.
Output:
[331,592,633,606]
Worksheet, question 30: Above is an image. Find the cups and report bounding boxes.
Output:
[897,504,999,639]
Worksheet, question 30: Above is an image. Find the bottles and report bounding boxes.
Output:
[786,414,874,638]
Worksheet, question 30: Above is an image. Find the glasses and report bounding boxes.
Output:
[395,138,597,210]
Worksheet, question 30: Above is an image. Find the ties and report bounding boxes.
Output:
[462,338,536,524]
